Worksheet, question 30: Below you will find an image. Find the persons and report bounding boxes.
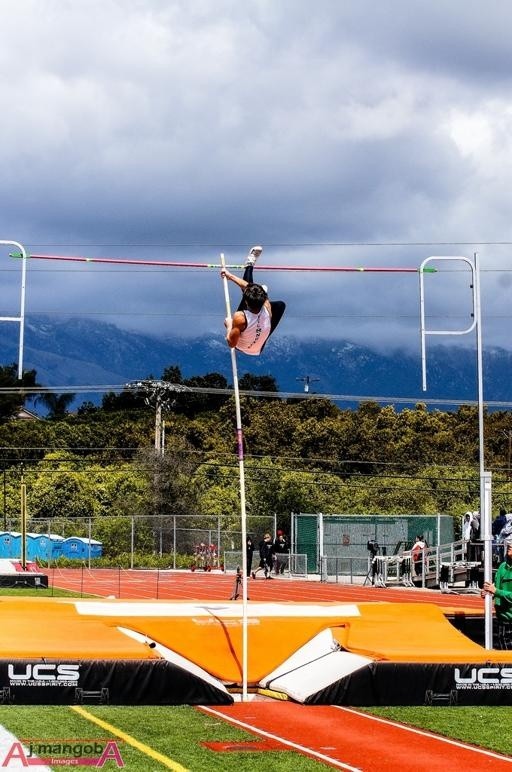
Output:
[246,537,255,577]
[222,244,287,358]
[263,535,278,579]
[497,514,512,563]
[270,535,289,574]
[491,508,508,540]
[401,534,427,576]
[479,542,512,652]
[251,533,274,579]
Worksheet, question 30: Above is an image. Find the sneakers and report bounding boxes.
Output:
[261,284,268,295]
[244,246,263,268]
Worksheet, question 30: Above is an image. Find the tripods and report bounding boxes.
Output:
[362,552,377,588]
